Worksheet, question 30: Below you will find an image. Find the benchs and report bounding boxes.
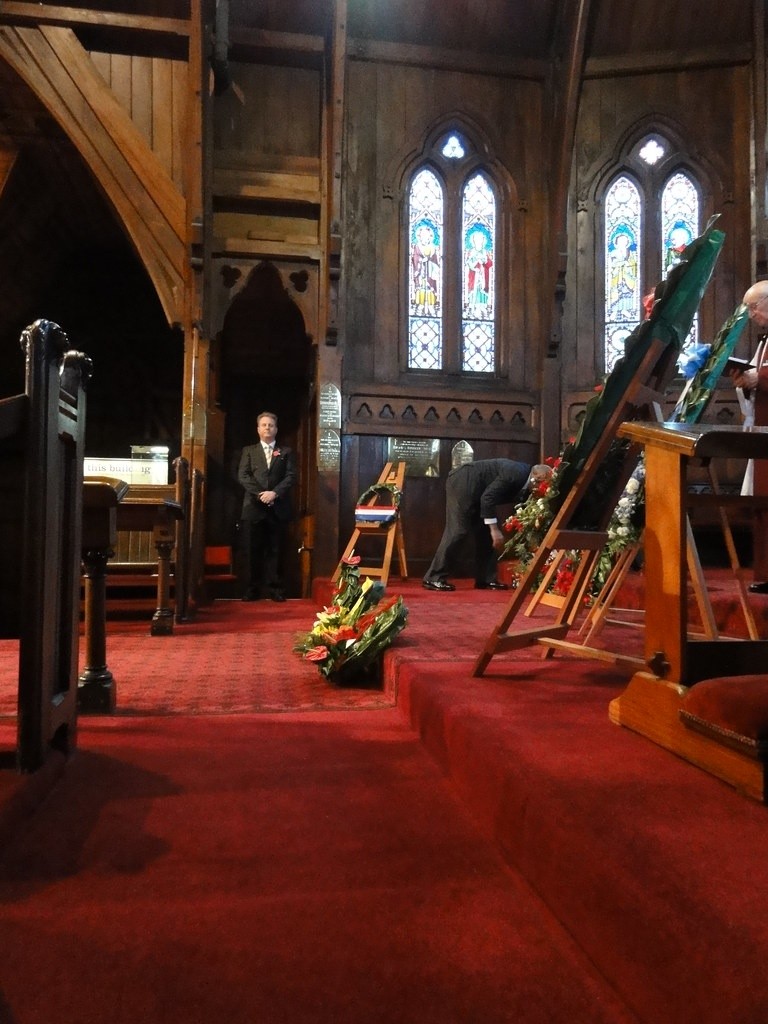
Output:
[103,457,189,625]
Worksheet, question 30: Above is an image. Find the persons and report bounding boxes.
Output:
[728,280,768,594]
[421,458,555,592]
[237,412,299,600]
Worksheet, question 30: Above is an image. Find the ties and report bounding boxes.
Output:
[265,445,272,468]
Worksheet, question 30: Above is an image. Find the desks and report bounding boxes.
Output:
[115,498,184,635]
[80,475,120,714]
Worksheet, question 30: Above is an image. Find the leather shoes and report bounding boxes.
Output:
[748,581,768,594]
[422,576,455,591]
[242,589,258,601]
[271,589,286,602]
[474,580,508,590]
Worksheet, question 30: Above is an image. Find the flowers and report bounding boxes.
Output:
[495,241,689,605]
[675,336,711,377]
[273,449,281,456]
[290,558,408,682]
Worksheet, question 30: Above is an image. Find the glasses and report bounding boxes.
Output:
[749,295,768,313]
